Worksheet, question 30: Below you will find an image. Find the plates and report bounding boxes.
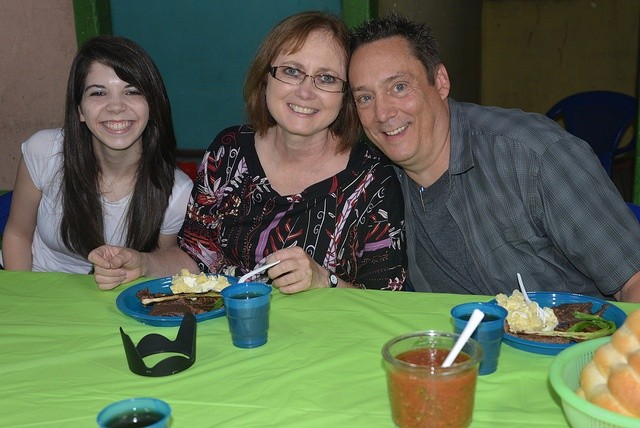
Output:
[485,290,628,356]
[115,272,250,327]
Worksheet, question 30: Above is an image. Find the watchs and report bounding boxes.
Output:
[326,266,339,288]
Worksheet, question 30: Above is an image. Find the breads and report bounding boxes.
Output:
[576,308,640,419]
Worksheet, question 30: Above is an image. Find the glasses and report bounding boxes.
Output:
[267,65,346,93]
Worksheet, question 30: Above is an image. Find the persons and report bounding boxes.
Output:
[87,11,407,295]
[1,35,194,273]
[344,13,640,304]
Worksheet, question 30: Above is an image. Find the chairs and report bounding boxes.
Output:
[544,91,638,181]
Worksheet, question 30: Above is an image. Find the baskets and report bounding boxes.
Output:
[549,336,639,428]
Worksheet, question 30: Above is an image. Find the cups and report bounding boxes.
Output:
[221,282,273,349]
[450,301,508,376]
[380,330,486,428]
[95,396,171,428]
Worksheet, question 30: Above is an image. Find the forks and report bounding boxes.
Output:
[514,272,546,324]
